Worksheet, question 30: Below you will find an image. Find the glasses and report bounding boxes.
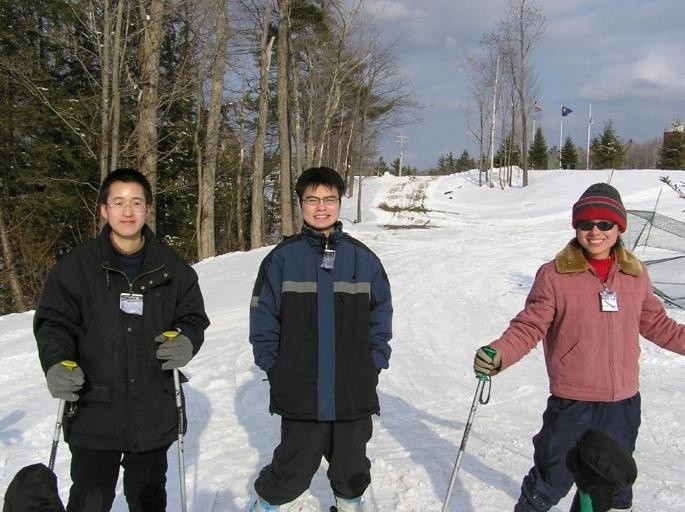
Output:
[103,201,148,212]
[298,197,340,207]
[577,222,614,231]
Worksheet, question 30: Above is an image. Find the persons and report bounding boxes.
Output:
[248,165,395,511]
[472,182,685,512]
[32,166,211,512]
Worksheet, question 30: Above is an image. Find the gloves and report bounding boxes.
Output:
[474,345,501,379]
[46,361,84,403]
[156,329,195,368]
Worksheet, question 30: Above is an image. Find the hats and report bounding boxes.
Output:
[572,184,628,233]
